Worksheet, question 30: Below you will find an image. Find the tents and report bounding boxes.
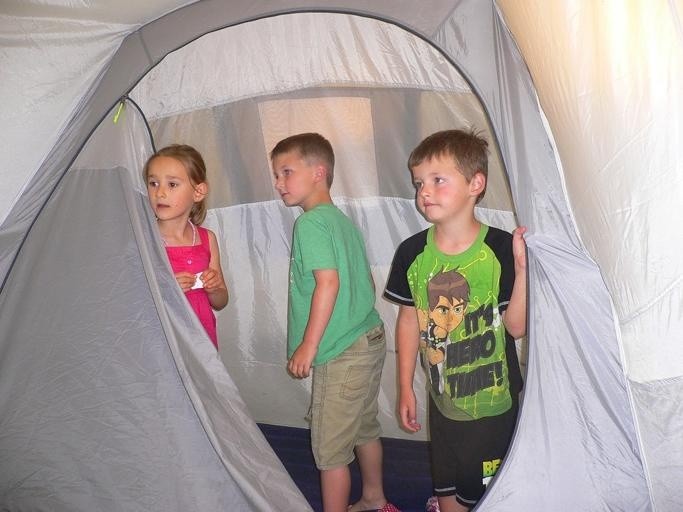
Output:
[2,1,680,512]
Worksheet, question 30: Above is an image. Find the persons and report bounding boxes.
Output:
[142,143,229,352]
[381,123,526,512]
[268,132,402,512]
